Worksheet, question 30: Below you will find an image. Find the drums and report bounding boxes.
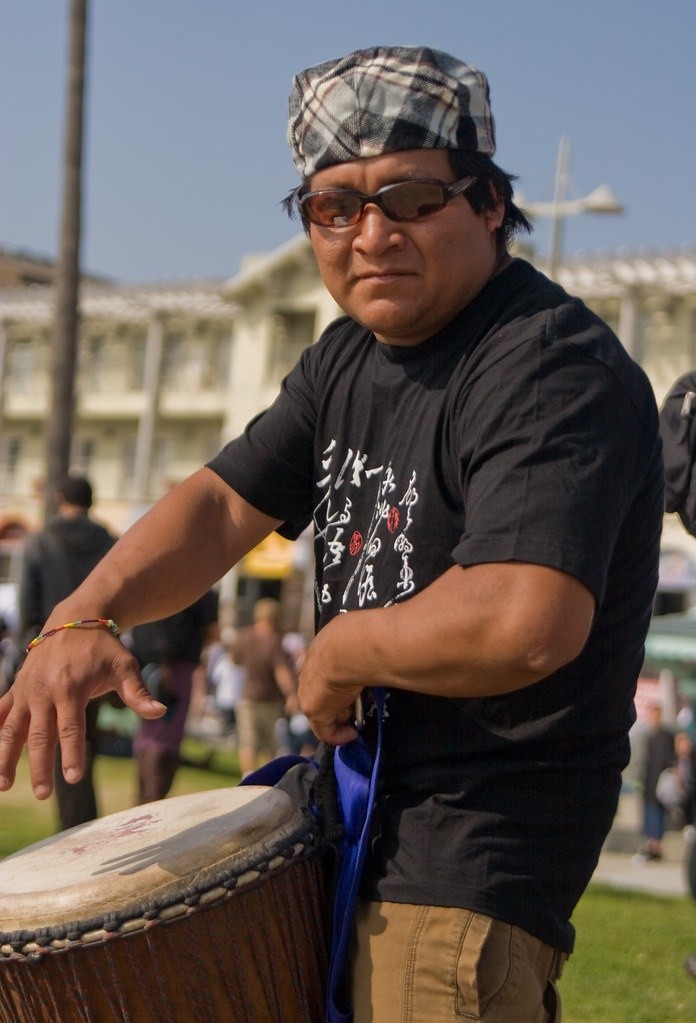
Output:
[0,784,328,1023]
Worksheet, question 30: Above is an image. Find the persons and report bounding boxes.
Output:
[625,663,695,980]
[1,477,321,840]
[1,44,663,1023]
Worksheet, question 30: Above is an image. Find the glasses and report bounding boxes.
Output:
[294,174,481,228]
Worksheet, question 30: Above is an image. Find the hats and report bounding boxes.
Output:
[287,45,496,179]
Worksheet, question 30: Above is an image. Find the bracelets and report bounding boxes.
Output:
[24,619,123,652]
